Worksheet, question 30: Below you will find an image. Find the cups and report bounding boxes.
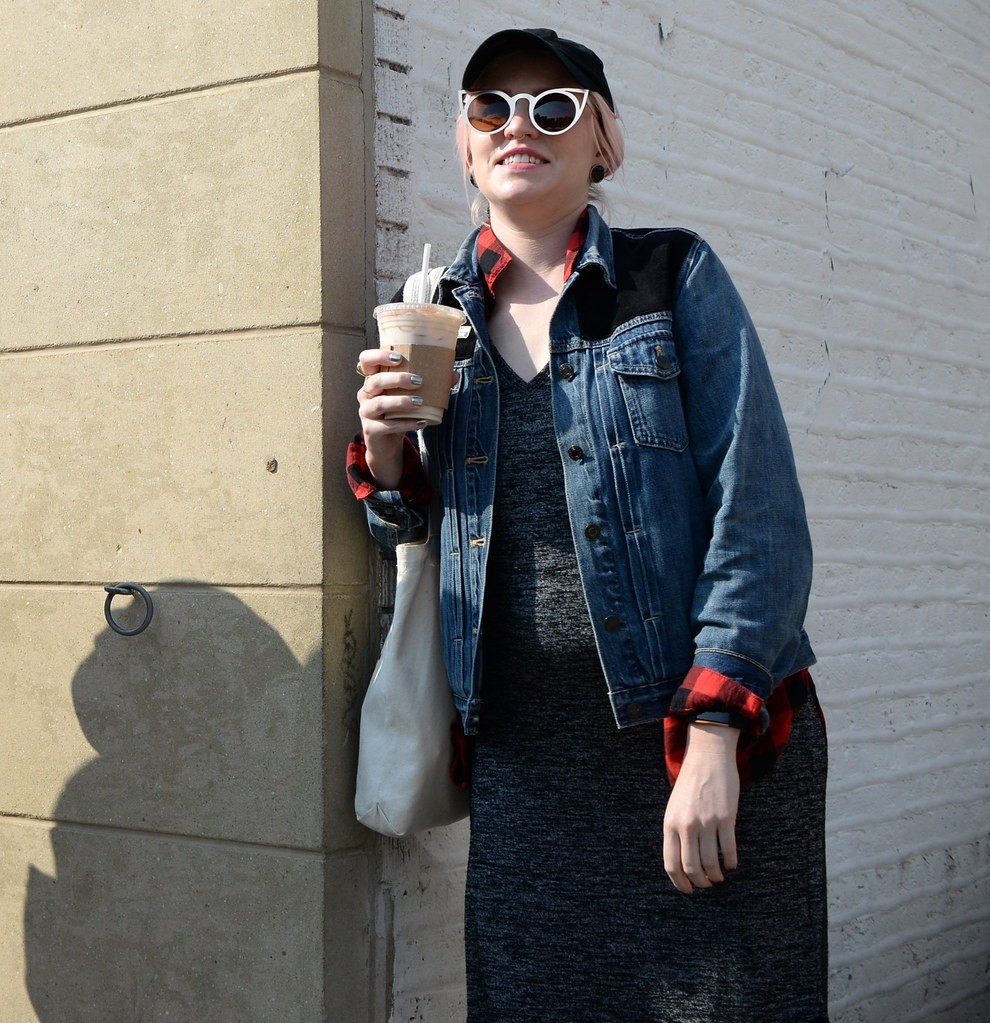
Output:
[373,303,469,429]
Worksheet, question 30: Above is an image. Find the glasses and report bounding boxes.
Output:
[455,85,601,140]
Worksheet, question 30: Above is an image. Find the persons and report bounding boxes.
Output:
[346,28,831,1023]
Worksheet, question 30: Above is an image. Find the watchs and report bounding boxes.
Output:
[683,707,744,728]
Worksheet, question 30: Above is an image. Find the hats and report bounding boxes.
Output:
[462,28,616,114]
